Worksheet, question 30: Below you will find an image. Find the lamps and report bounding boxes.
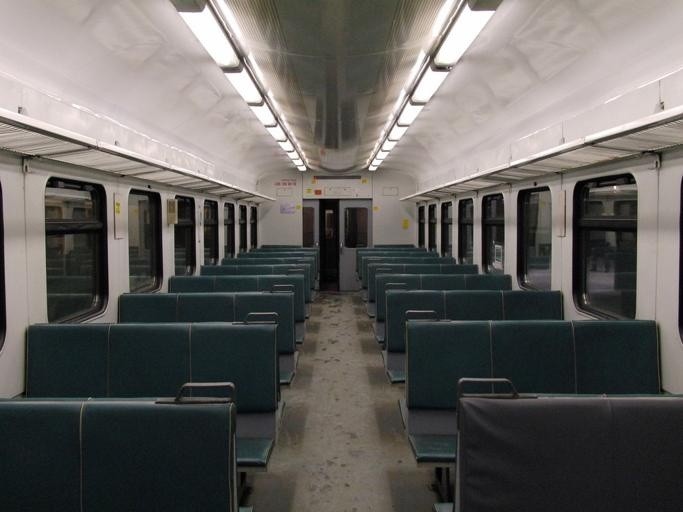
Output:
[367,0,499,172]
[164,0,308,171]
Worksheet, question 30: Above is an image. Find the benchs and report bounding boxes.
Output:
[350,245,682,511]
[1,244,319,512]
